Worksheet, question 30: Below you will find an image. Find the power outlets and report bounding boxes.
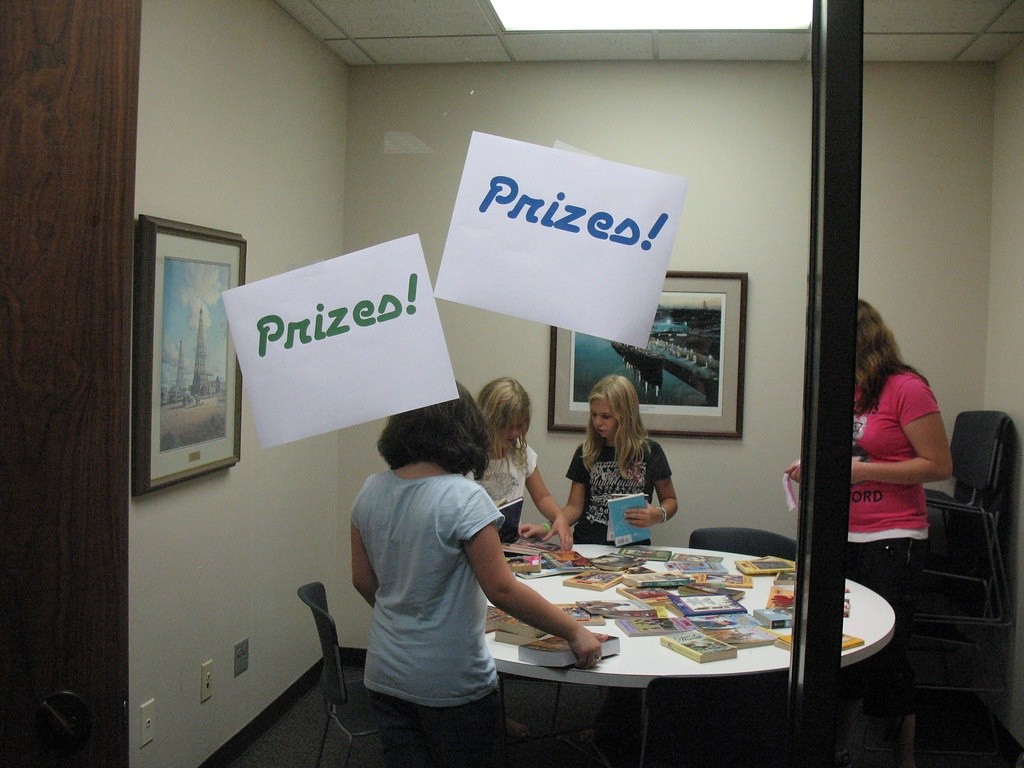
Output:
[235,637,249,677]
[201,660,214,704]
[140,699,154,748]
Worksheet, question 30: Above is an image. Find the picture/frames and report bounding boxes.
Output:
[546,271,749,444]
[132,214,247,498]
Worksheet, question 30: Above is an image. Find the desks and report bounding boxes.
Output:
[485,543,896,768]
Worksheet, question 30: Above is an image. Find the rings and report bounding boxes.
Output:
[594,655,602,662]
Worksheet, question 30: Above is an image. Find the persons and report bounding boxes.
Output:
[786,299,954,768]
[520,376,679,741]
[350,378,608,768]
[464,378,575,741]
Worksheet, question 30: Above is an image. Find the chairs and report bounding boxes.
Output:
[296,580,383,768]
[690,412,1024,768]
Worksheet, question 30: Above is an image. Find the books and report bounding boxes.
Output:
[476,534,865,668]
[604,490,651,548]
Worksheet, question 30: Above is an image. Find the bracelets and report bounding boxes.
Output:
[654,504,670,530]
[541,521,553,540]
